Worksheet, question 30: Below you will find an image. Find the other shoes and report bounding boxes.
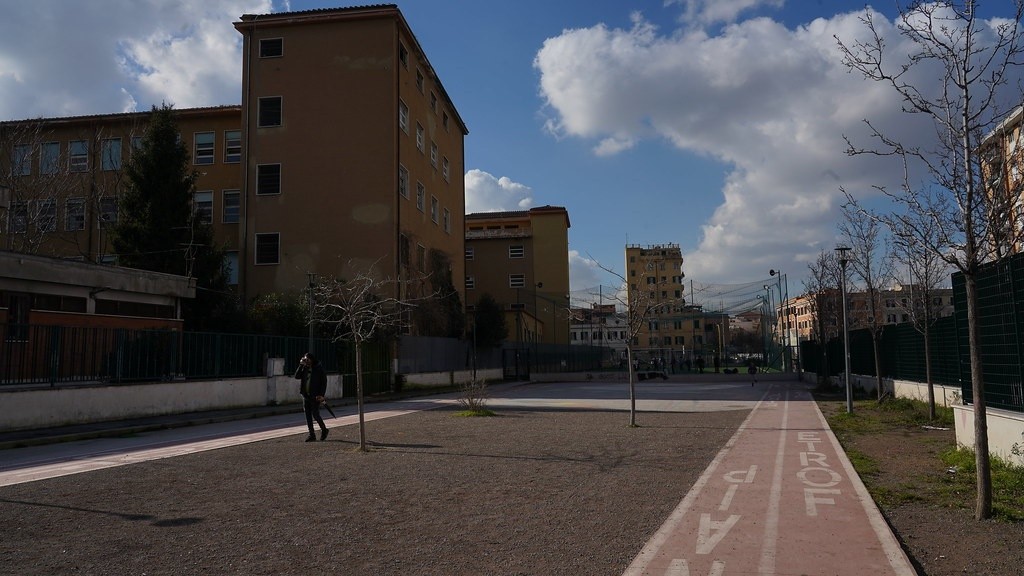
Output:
[305,437,316,442]
[320,429,329,441]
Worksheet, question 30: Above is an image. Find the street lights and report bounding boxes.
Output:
[833,248,854,415]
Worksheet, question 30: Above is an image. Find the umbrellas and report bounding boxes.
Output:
[316,396,337,420]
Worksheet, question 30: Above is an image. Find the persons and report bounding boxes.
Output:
[748,362,758,387]
[295,353,330,442]
